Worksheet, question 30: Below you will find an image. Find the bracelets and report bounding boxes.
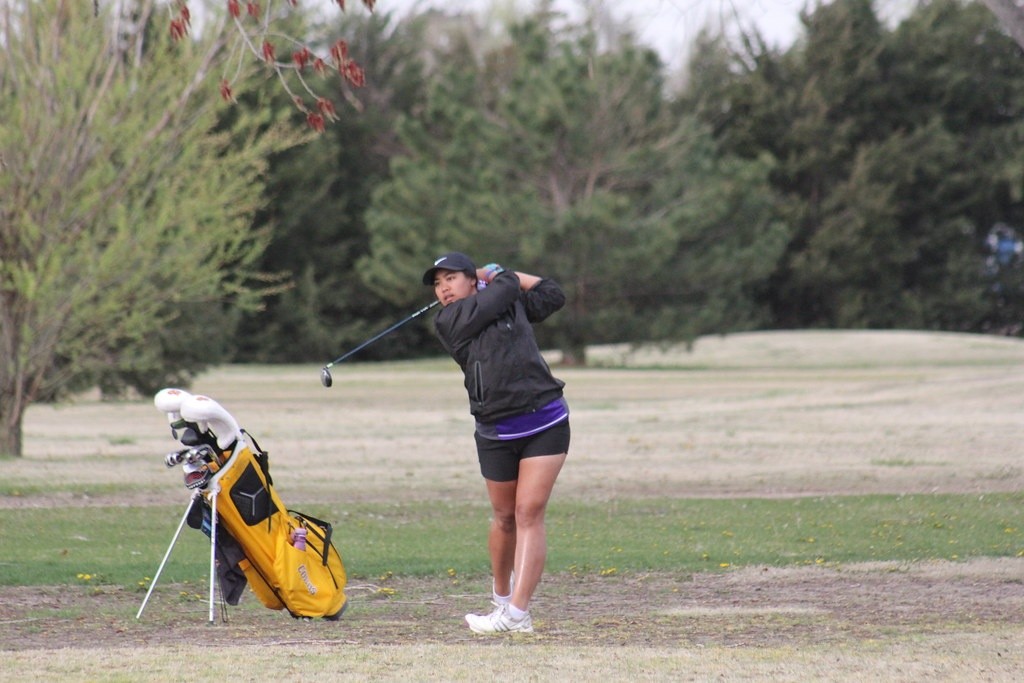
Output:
[485,269,497,280]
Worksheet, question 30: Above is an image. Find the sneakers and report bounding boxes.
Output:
[492,570,514,608]
[464,605,534,635]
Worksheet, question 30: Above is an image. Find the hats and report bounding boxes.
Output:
[423,252,479,285]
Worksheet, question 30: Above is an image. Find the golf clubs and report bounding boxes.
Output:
[154,388,242,490]
[321,299,442,388]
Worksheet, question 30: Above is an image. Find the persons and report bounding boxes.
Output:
[422,252,570,635]
